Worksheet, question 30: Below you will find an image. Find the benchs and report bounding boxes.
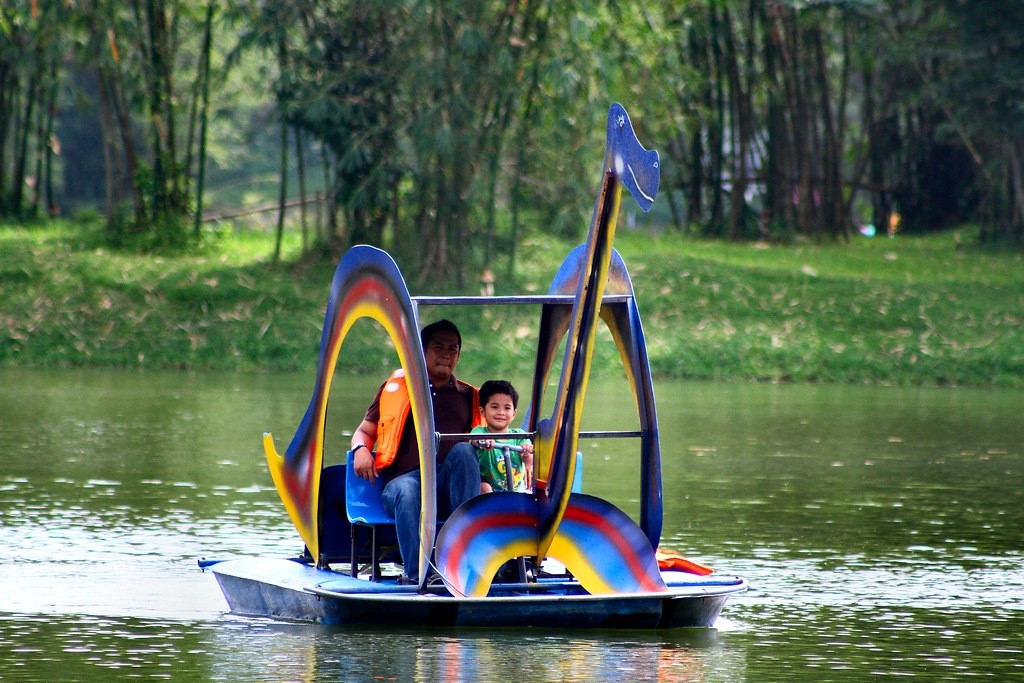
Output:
[346,450,447,582]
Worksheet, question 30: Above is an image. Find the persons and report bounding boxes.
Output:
[469,380,534,494]
[351,320,488,580]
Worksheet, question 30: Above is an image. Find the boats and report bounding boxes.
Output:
[196,97,748,641]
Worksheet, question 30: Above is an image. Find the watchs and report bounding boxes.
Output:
[351,444,367,454]
